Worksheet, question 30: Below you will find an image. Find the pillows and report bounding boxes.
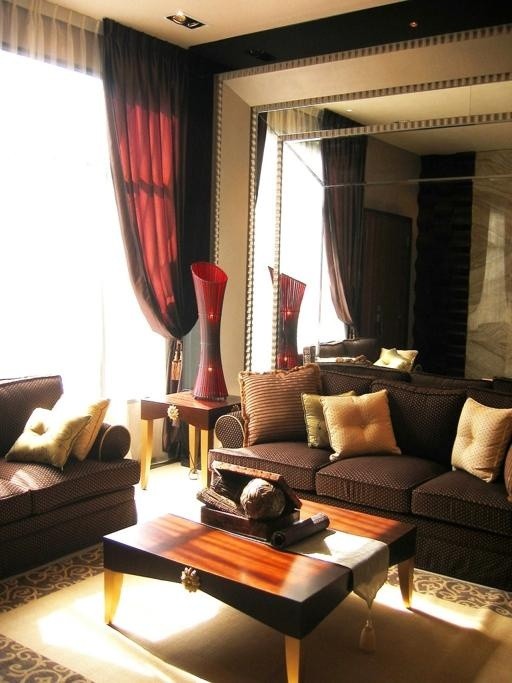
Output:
[5,391,113,469]
[374,347,418,373]
[450,396,511,484]
[236,366,403,458]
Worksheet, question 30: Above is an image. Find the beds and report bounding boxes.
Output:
[301,336,382,362]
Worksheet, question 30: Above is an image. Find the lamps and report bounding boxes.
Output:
[267,265,306,374]
[191,261,231,400]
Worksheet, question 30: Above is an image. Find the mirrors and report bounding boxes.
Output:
[240,70,512,373]
[272,115,512,372]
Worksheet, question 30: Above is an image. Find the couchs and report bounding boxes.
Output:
[0,372,142,581]
[208,371,511,595]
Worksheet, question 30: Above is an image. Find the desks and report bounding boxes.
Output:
[139,389,243,491]
